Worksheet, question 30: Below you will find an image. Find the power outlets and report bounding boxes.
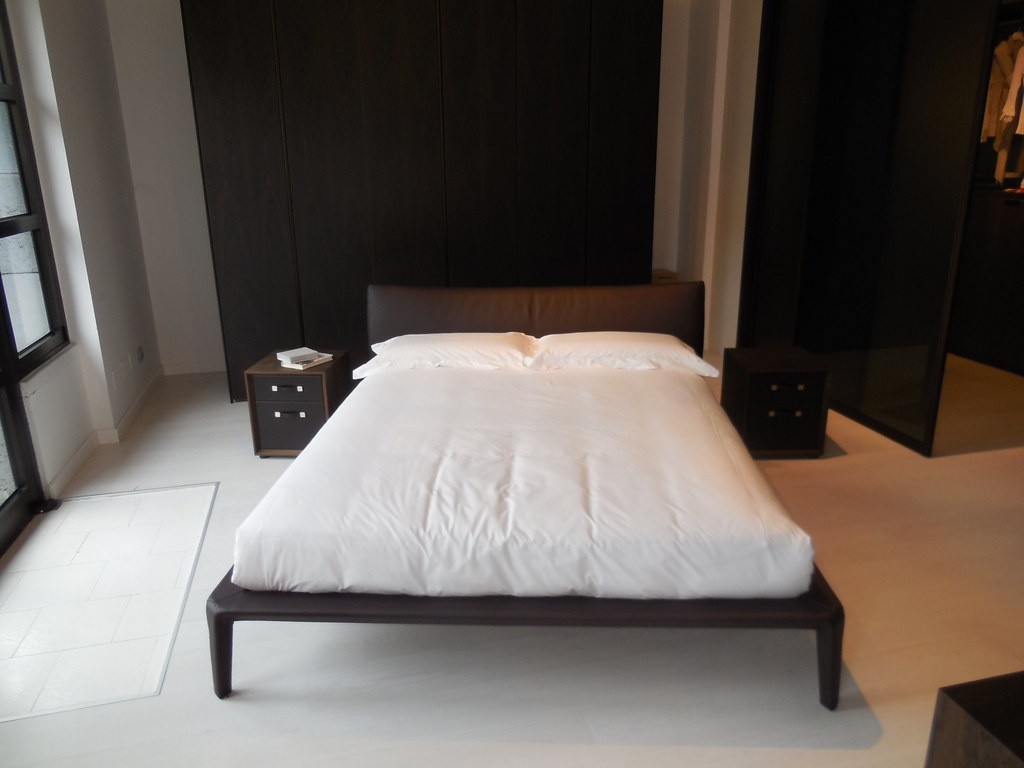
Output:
[112,346,145,390]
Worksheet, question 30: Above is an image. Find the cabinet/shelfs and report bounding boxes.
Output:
[180,0,666,402]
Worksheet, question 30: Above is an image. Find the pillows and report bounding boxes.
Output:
[352,331,536,381]
[533,332,719,378]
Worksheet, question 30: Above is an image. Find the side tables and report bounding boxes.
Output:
[721,348,833,458]
[243,348,352,459]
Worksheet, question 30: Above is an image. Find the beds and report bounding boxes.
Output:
[206,278,845,710]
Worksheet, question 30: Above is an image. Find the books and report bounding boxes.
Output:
[276,346,333,370]
